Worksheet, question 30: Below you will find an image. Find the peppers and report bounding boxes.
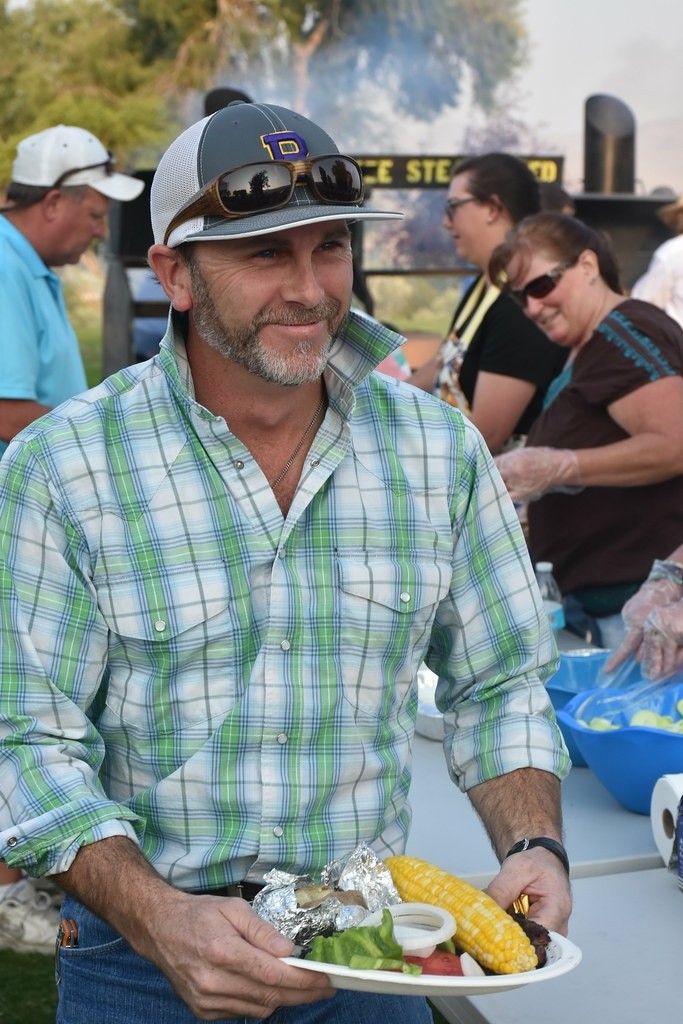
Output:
[437,939,456,954]
[302,908,421,974]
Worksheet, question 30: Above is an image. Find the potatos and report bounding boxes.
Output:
[292,885,368,910]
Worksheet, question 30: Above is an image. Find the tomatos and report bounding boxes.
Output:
[402,950,463,975]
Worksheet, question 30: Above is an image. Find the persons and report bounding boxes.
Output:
[482,211,683,649]
[602,545,683,679]
[397,151,554,448]
[0,99,573,1024]
[629,195,683,326]
[0,122,145,463]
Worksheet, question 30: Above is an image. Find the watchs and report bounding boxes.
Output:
[503,837,569,879]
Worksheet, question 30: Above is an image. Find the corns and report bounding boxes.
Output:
[382,855,537,974]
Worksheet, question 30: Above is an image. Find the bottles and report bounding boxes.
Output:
[533,563,566,644]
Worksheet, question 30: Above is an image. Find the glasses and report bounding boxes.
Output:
[443,197,487,221]
[51,150,115,191]
[163,152,364,246]
[507,257,579,309]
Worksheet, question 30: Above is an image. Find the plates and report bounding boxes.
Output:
[275,924,581,998]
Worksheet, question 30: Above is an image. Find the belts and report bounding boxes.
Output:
[185,881,264,903]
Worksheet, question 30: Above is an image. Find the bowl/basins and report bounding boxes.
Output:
[538,653,683,814]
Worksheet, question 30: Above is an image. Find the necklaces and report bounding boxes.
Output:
[269,391,328,492]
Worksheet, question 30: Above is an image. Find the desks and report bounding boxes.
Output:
[399,726,683,1024]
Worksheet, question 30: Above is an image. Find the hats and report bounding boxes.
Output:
[12,124,144,201]
[150,99,406,249]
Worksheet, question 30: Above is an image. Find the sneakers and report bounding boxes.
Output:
[0,885,61,954]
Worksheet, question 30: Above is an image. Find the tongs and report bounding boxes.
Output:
[576,649,683,719]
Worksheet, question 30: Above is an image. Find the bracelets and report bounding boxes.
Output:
[649,559,683,585]
